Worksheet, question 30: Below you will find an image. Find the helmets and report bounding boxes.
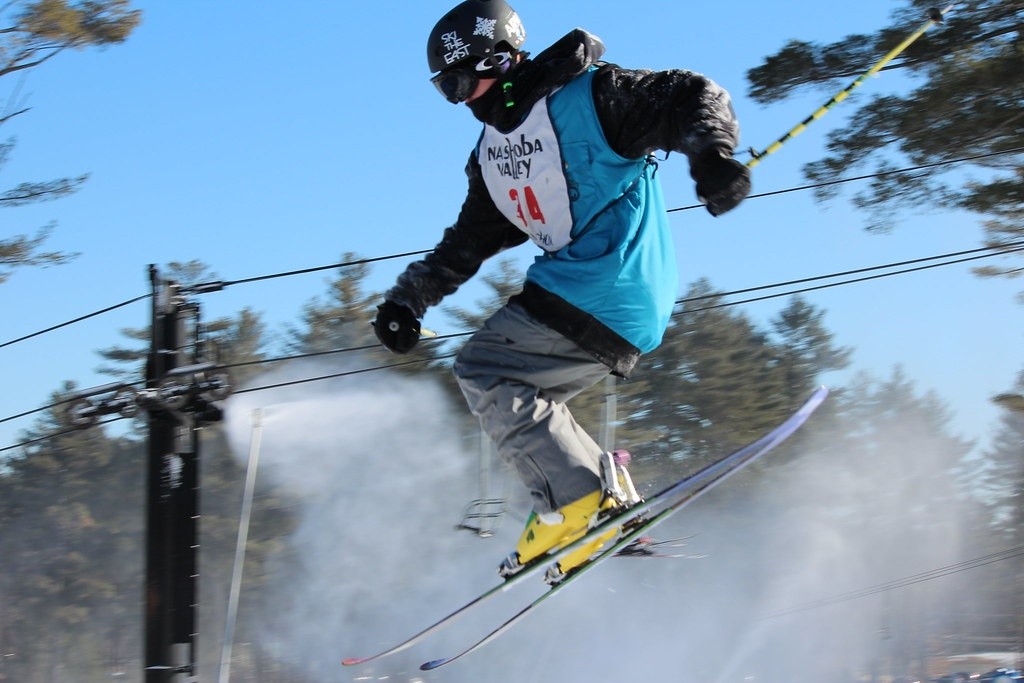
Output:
[427,0,527,72]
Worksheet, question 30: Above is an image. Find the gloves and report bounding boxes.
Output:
[695,155,751,217]
[372,302,420,354]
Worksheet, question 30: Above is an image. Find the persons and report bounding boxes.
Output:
[373,0,750,584]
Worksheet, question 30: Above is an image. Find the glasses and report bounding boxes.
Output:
[430,69,479,104]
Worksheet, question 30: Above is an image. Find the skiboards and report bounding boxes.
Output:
[342,384,827,673]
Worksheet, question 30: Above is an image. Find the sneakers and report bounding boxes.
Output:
[498,488,620,579]
[543,524,621,586]
